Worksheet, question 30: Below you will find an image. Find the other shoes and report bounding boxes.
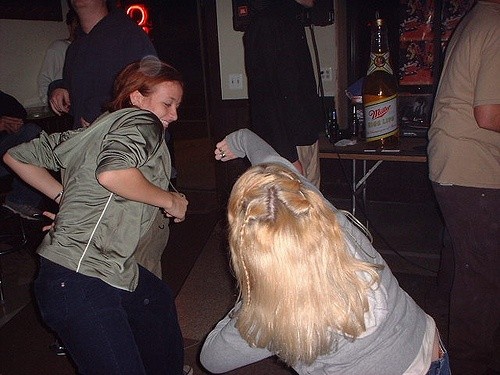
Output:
[1,199,49,220]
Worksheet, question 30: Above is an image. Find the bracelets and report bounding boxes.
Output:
[54,190,63,202]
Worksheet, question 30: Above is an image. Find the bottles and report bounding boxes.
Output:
[351,105,359,138]
[326,107,340,143]
[362,17,399,148]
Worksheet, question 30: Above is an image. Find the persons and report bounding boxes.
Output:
[242,0,329,191]
[2,59,189,375]
[0,0,171,357]
[428,0,500,375]
[199,127,452,375]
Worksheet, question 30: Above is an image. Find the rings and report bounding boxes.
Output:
[220,152,226,158]
[219,147,223,153]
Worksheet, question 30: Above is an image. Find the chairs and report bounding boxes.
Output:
[0,185,35,305]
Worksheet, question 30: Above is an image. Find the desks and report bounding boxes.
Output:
[24,108,70,134]
[318,133,429,223]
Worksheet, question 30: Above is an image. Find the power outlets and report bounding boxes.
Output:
[230,74,242,89]
[321,67,332,81]
